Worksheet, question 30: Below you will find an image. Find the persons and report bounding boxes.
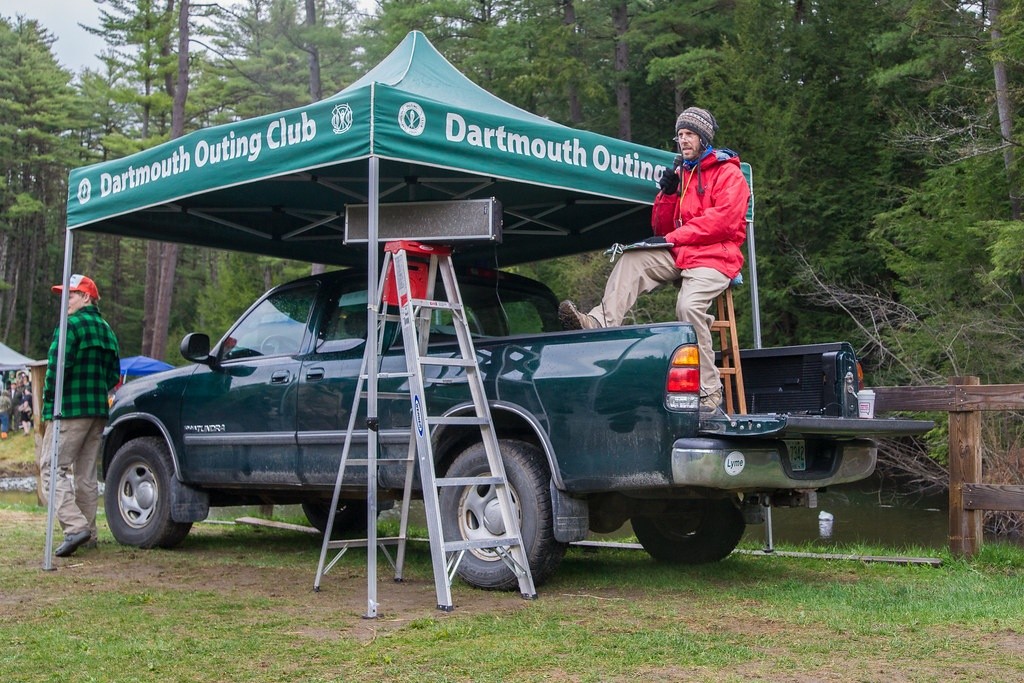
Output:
[559,107,751,419]
[0,370,34,439]
[40,273,120,556]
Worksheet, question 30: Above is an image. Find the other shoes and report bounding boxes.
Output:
[558,300,601,330]
[699,387,722,417]
[54,530,91,556]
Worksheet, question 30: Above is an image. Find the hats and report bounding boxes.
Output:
[675,106,719,144]
[52,273,98,300]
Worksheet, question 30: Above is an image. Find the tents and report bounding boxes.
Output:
[0,342,49,370]
[120,353,176,385]
[42,30,775,621]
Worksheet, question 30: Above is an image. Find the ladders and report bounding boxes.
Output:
[311,238,540,610]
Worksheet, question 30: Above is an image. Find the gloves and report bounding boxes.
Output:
[659,167,680,195]
[643,237,666,243]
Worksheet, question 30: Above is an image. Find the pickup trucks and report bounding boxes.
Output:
[97,262,937,592]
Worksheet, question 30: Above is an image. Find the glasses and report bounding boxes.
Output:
[672,133,699,143]
